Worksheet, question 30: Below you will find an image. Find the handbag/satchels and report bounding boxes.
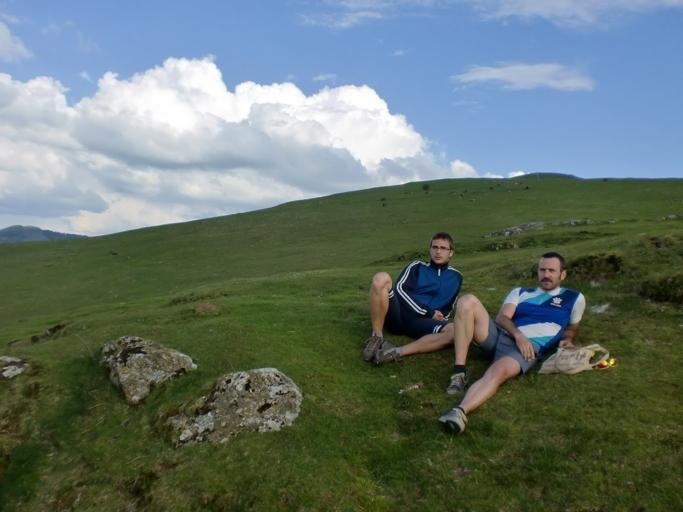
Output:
[536,343,609,375]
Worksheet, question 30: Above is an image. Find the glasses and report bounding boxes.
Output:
[430,244,452,251]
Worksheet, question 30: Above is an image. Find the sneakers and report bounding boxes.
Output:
[362,333,384,362]
[446,372,466,395]
[374,340,400,368]
[437,407,468,435]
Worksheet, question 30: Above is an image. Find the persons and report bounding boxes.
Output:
[436,251,586,435]
[358,232,464,368]
